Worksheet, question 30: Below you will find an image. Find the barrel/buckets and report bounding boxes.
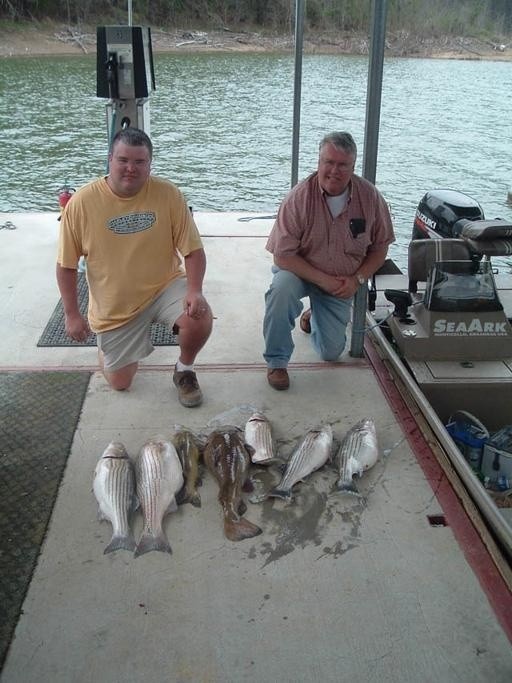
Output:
[445,408,489,476]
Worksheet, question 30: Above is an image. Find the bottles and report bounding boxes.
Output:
[481,474,512,489]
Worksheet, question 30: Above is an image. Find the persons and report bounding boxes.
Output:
[54,127,214,407]
[260,128,397,390]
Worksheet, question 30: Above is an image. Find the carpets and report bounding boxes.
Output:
[37,272,179,346]
[1,370,92,672]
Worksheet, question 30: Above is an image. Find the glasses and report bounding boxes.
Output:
[111,155,151,168]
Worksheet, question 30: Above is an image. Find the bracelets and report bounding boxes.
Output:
[354,272,365,287]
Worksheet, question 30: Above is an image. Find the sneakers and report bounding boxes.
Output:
[172,361,204,408]
[266,366,290,391]
[298,305,317,334]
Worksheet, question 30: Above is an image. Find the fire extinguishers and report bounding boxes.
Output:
[55,184,76,221]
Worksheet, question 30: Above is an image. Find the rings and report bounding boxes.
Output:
[201,308,206,312]
